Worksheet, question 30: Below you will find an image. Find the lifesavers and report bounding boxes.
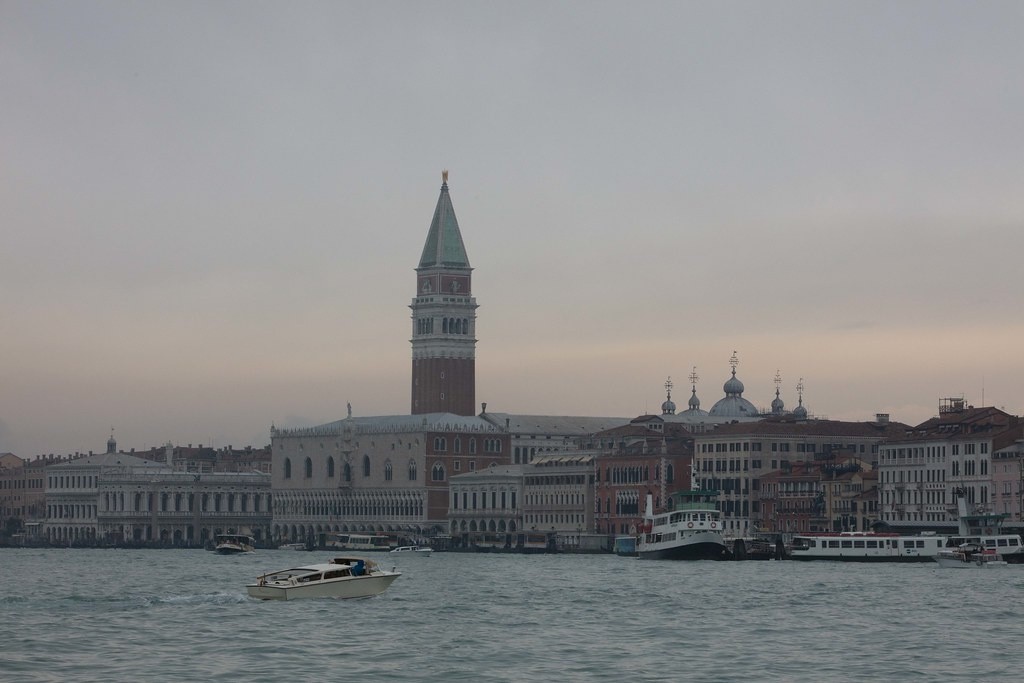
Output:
[711,522,716,528]
[977,506,984,513]
[688,521,693,528]
[986,528,992,535]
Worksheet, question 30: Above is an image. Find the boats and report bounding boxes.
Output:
[332,533,392,552]
[278,542,309,551]
[215,525,256,555]
[787,528,1024,561]
[244,556,402,602]
[633,456,731,561]
[389,545,435,557]
[932,539,1009,568]
